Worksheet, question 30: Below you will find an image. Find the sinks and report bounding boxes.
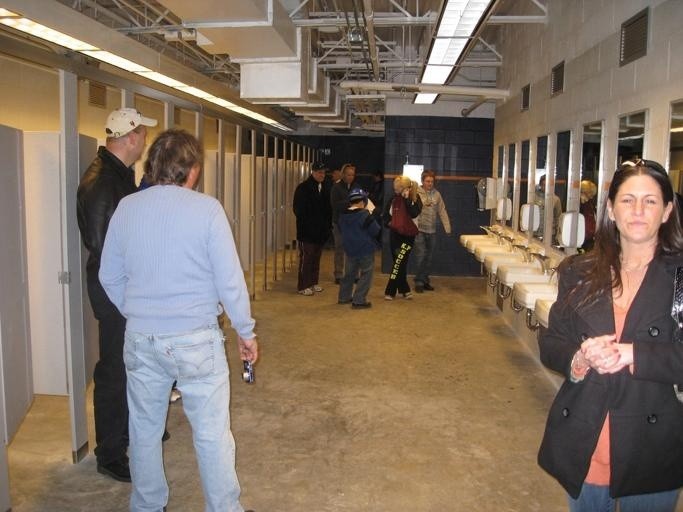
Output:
[460,224,562,330]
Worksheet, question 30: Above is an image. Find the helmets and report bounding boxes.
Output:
[348,187,368,206]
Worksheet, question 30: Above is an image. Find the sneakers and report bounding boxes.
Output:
[404,282,433,299]
[384,293,392,301]
[97,454,131,481]
[313,285,322,291]
[352,300,371,309]
[298,287,312,295]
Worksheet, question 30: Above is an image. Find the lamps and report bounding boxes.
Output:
[0,0,297,131]
[411,0,499,104]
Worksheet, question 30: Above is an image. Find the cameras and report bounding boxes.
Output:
[242,360,254,383]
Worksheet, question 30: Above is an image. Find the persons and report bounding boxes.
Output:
[537,159,683,512]
[98,128,259,510]
[579,180,597,254]
[533,174,562,243]
[412,170,452,293]
[76,108,170,484]
[383,175,423,301]
[329,164,384,309]
[292,161,332,296]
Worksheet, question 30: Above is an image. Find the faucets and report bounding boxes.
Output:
[480,225,560,279]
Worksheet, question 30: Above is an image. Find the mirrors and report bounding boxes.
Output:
[498,98,683,257]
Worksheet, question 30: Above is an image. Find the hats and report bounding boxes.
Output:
[312,162,329,171]
[105,108,158,138]
